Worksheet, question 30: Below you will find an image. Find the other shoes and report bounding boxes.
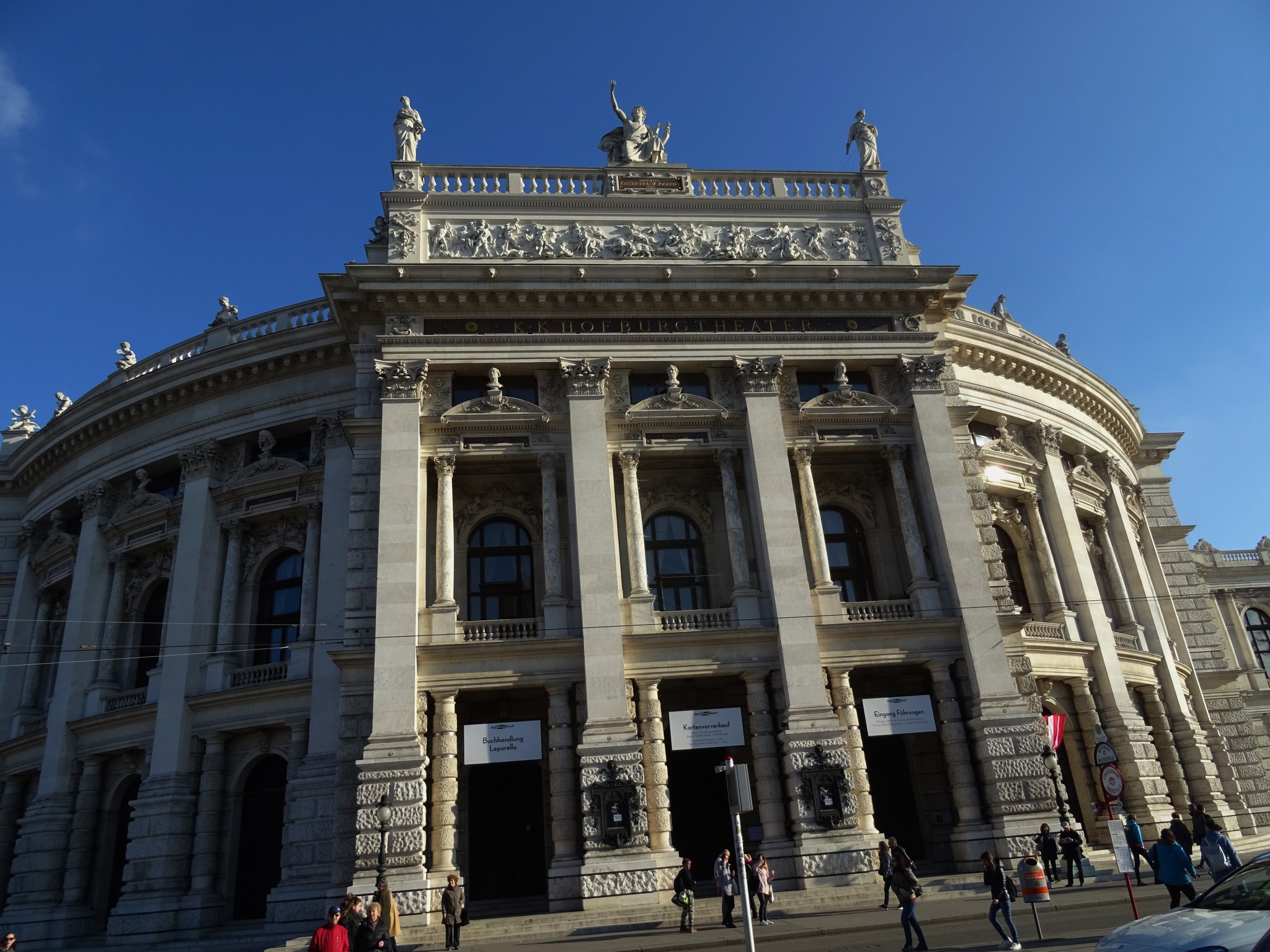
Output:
[1065,883,1084,887]
[1194,864,1213,878]
[901,943,928,952]
[455,946,460,950]
[1049,878,1061,882]
[1137,879,1164,886]
[448,947,452,950]
[752,914,774,925]
[721,920,737,928]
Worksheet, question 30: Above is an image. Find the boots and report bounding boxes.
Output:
[680,924,697,933]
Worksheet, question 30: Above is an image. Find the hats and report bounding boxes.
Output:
[1062,821,1069,828]
[329,906,340,912]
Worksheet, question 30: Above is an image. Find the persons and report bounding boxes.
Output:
[888,235,895,246]
[610,79,670,164]
[307,872,466,952]
[845,110,880,170]
[1120,476,1132,493]
[393,96,425,161]
[996,416,1008,434]
[55,392,72,415]
[673,804,1249,952]
[208,296,239,325]
[665,364,680,384]
[0,931,16,952]
[7,405,35,429]
[135,468,151,487]
[1055,333,1071,355]
[991,294,1007,318]
[834,362,849,382]
[1073,443,1088,461]
[486,367,503,388]
[431,219,868,256]
[368,216,389,243]
[399,230,410,242]
[50,509,63,528]
[116,342,136,368]
[259,430,275,453]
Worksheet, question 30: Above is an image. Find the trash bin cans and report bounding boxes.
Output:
[1017,857,1052,903]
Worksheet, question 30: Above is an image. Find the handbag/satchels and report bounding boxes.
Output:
[767,882,774,903]
[1035,834,1043,851]
[902,867,923,898]
[731,878,738,895]
[460,902,470,927]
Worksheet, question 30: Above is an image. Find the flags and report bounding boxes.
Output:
[1043,714,1067,750]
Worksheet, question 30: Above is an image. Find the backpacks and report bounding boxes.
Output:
[1124,825,1136,848]
[1201,835,1232,872]
[671,869,689,907]
[994,866,1018,902]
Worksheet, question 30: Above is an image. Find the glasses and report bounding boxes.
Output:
[328,914,340,917]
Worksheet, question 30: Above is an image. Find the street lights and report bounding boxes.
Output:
[1040,744,1071,827]
[372,791,393,902]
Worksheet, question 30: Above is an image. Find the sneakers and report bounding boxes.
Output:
[878,904,903,910]
[998,936,1022,950]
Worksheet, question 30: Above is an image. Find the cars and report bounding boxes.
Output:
[1094,850,1270,952]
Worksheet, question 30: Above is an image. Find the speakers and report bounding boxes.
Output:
[725,763,753,815]
